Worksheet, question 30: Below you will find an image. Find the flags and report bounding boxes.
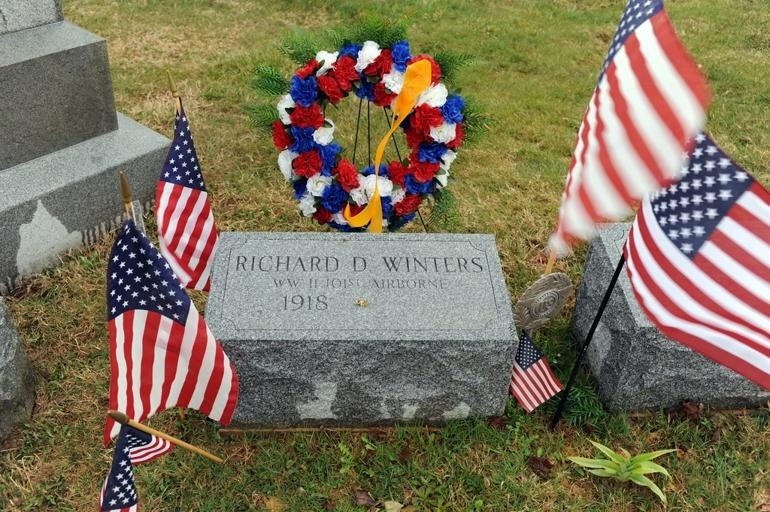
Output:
[511,332,565,418]
[156,103,219,292]
[621,128,770,392]
[551,4,713,262]
[103,218,240,450]
[97,424,175,512]
